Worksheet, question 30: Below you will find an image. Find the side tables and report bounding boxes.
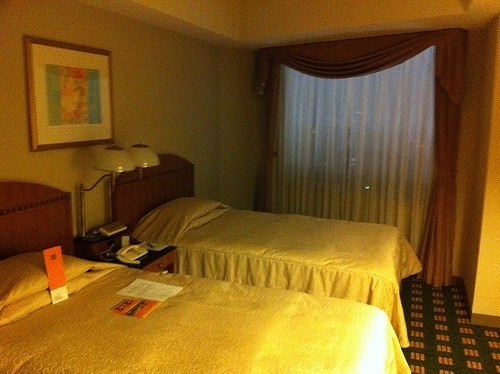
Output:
[76,222,179,273]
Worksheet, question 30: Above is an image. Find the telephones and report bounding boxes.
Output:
[117,244,148,262]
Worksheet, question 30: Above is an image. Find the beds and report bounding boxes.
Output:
[0,182,413,373]
[113,154,423,350]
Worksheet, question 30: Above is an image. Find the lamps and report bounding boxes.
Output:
[79,145,160,236]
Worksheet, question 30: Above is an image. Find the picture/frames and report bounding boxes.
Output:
[24,37,116,150]
